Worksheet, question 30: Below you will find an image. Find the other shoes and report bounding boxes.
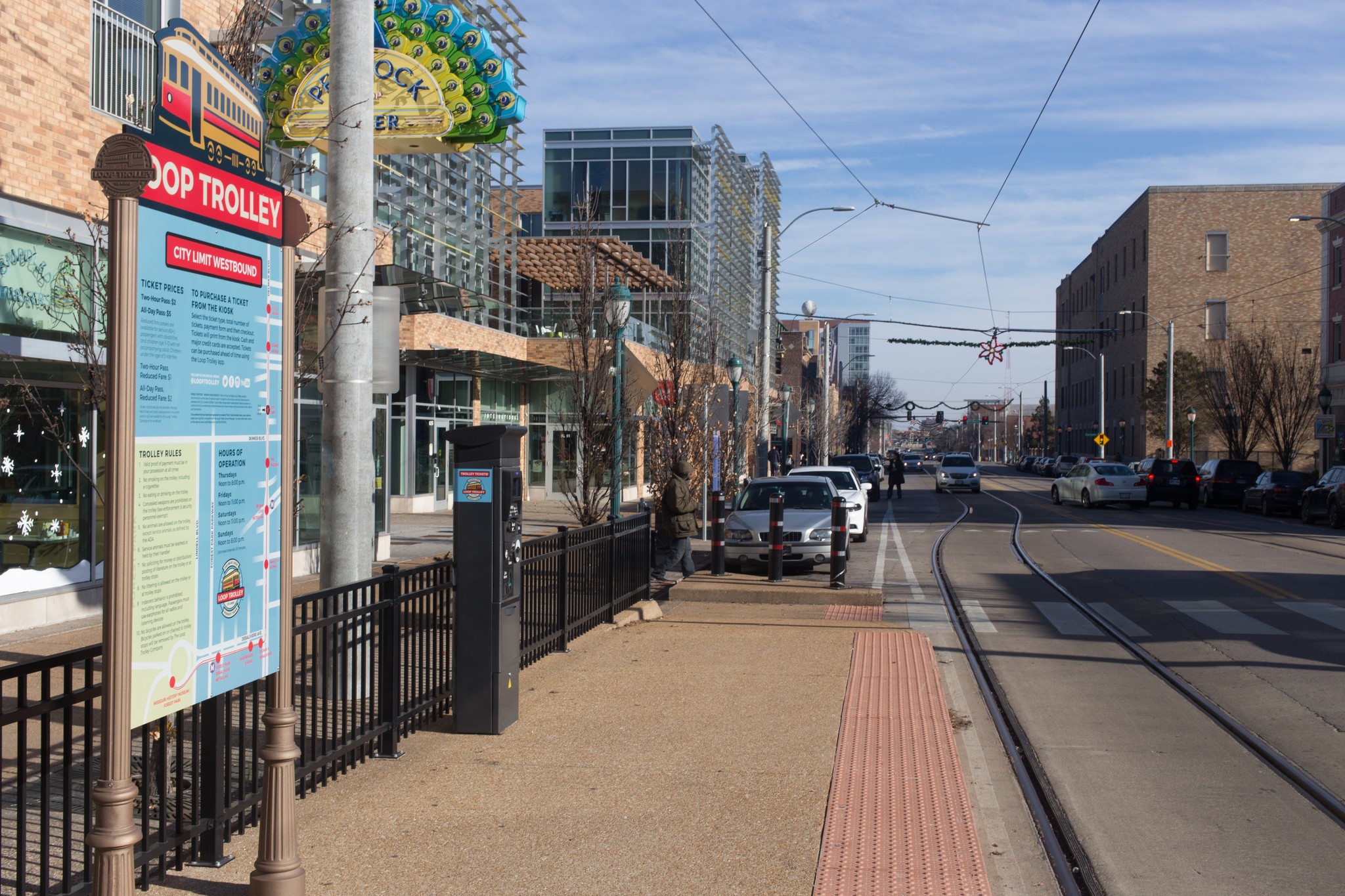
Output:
[886,497,892,500]
[897,496,902,499]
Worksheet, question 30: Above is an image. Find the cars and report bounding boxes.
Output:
[787,466,873,541]
[1016,455,1105,475]
[830,451,883,501]
[725,477,854,571]
[1050,463,1146,508]
[886,450,972,471]
[1194,459,1345,528]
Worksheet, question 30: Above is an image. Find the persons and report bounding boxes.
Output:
[650,461,700,584]
[768,445,781,476]
[799,450,831,467]
[786,452,795,474]
[884,452,905,498]
[1112,451,1122,462]
[868,449,873,454]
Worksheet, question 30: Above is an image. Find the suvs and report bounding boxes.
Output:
[1128,458,1198,510]
[935,453,982,494]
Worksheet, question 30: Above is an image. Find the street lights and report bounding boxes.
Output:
[806,396,818,467]
[1064,346,1105,460]
[1317,384,1332,475]
[838,353,874,454]
[1187,406,1197,463]
[603,276,635,521]
[726,353,745,512]
[775,382,791,475]
[757,206,854,476]
[1118,310,1175,460]
[823,313,876,466]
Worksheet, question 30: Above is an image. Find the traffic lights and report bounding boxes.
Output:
[936,411,944,423]
[982,416,989,425]
[908,411,912,420]
[963,416,967,425]
[1099,435,1104,445]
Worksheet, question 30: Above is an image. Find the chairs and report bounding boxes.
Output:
[757,487,824,511]
